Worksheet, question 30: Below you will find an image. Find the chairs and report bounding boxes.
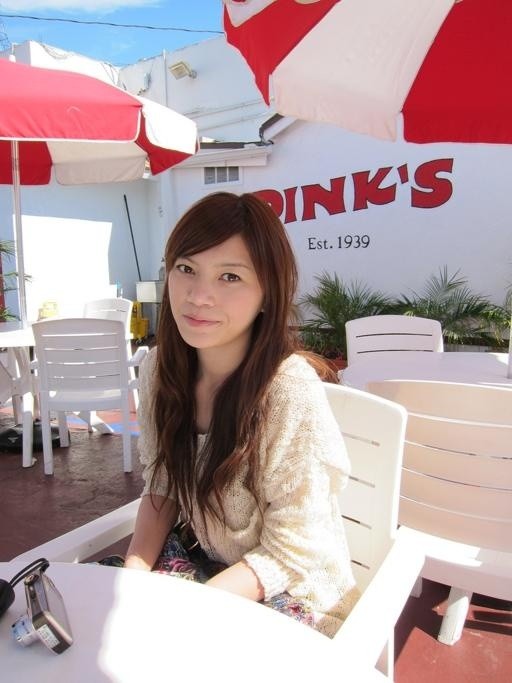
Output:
[11,382,426,681]
[366,378,511,645]
[85,296,140,412]
[346,313,445,361]
[33,317,150,476]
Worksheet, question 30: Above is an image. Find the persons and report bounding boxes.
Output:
[71,191,362,641]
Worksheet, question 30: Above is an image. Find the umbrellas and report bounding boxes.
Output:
[217,0,510,145]
[0,55,202,322]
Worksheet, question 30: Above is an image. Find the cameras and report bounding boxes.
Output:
[11,567,74,655]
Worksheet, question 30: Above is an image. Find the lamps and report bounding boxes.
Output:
[168,59,197,80]
